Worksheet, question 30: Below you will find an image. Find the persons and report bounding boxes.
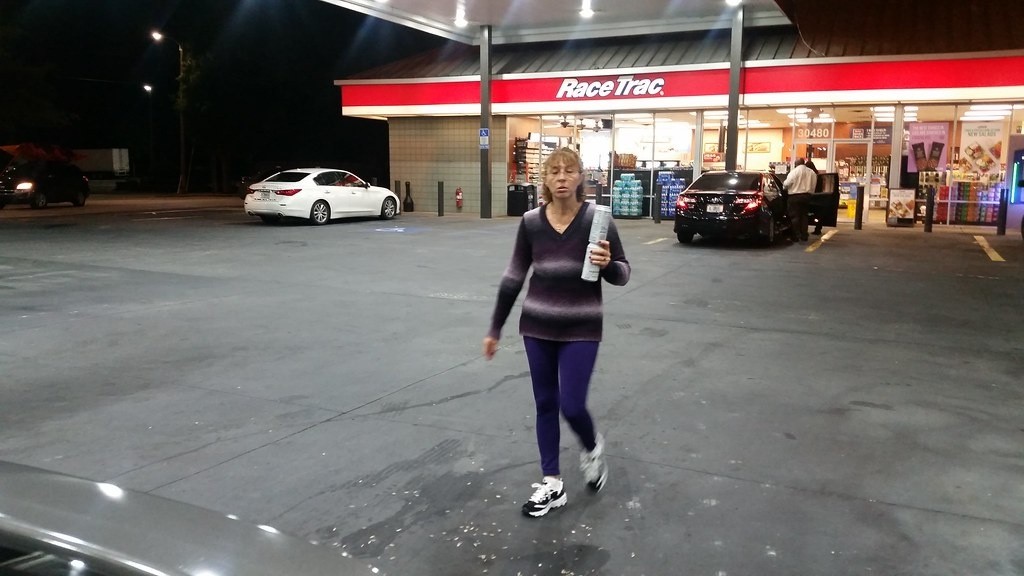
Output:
[479,147,633,518]
[781,157,817,244]
[802,160,823,234]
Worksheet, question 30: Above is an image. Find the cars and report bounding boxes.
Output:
[674,167,841,249]
[244,166,402,227]
[0,145,93,208]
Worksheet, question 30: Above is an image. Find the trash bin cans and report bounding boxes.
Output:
[507,184,535,216]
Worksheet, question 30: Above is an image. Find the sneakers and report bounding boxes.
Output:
[521,475,568,519]
[581,434,607,491]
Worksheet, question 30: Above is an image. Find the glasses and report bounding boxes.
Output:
[545,168,579,178]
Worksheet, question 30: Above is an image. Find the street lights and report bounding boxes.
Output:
[140,29,191,196]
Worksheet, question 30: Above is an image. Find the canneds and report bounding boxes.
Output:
[581,205,612,281]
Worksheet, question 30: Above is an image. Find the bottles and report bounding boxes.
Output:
[613,172,644,216]
[657,170,686,215]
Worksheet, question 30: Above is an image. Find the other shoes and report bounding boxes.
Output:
[791,233,808,242]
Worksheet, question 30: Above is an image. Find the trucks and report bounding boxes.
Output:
[70,148,132,179]
